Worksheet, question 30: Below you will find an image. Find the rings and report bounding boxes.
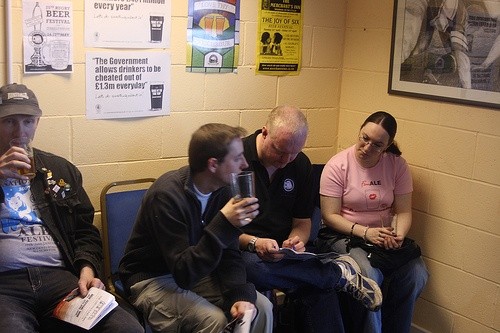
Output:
[399,244,401,249]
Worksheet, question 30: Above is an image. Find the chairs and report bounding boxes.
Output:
[100,164,326,333]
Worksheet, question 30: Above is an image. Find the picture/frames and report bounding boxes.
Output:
[386,0,500,108]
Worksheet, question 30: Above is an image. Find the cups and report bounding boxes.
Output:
[228,171,255,204]
[379,213,399,242]
[9,137,37,180]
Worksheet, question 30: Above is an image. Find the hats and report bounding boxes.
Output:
[0,83,42,118]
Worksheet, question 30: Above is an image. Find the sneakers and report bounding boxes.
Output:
[330,256,383,311]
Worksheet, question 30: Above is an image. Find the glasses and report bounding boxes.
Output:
[358,136,393,153]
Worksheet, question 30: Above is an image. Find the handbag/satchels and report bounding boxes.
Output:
[317,227,422,274]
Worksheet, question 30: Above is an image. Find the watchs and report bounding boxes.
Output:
[248,237,257,252]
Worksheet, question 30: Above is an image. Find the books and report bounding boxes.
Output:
[51,286,118,330]
[278,248,341,261]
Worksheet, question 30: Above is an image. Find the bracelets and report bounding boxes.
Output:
[363,226,369,240]
[350,223,358,235]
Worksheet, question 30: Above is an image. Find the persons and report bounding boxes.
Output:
[0,83,145,333]
[260,31,283,55]
[315,111,428,333]
[118,123,273,333]
[237,104,382,333]
